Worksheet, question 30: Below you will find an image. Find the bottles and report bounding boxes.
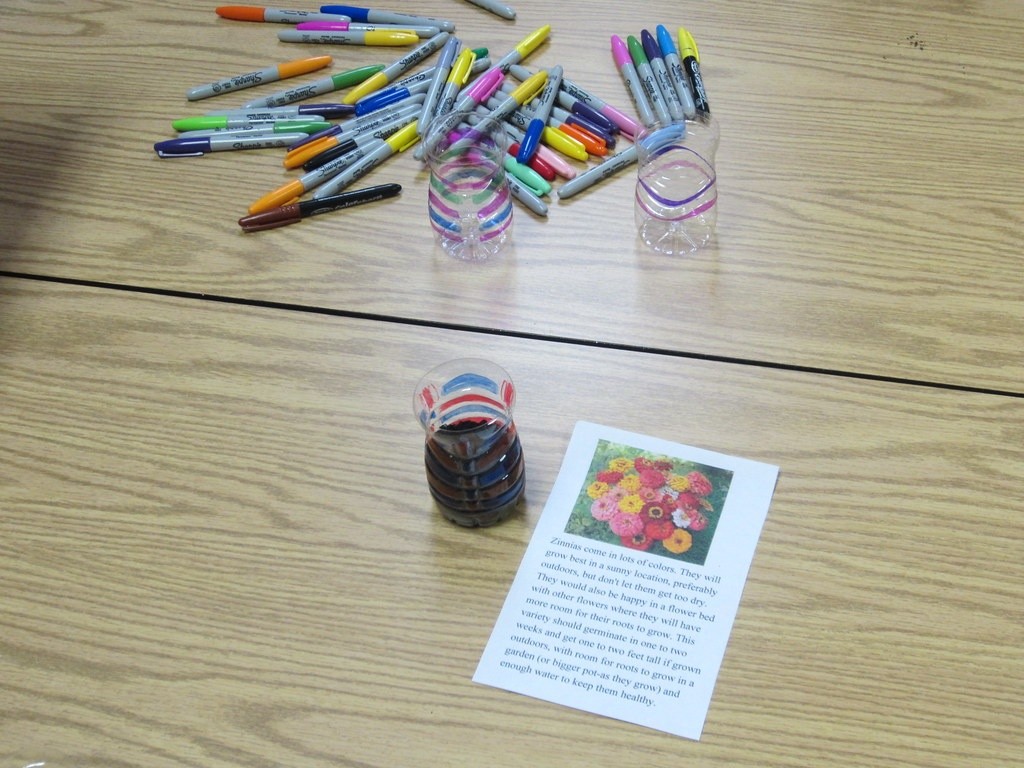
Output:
[413,359,527,529]
[423,111,515,261]
[633,108,721,258]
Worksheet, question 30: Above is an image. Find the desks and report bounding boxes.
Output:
[1,0,1024,768]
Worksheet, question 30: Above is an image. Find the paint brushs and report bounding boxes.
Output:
[153,0,713,234]
